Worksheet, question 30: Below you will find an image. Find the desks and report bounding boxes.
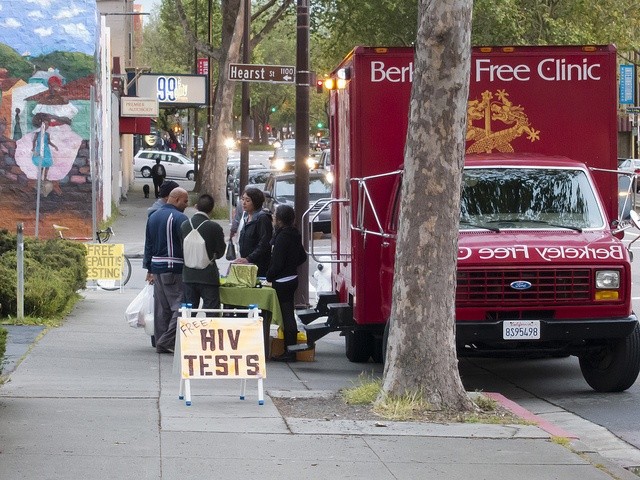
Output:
[218,283,273,361]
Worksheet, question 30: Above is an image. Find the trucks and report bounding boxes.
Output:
[300,40,639,394]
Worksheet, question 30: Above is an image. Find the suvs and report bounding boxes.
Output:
[132,150,200,181]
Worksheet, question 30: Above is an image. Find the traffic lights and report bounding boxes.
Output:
[316,122,324,128]
[267,126,270,131]
[316,80,323,94]
[316,132,322,138]
[271,107,276,112]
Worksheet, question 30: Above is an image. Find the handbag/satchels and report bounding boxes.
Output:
[226,239,236,260]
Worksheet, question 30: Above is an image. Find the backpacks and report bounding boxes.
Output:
[183,217,215,270]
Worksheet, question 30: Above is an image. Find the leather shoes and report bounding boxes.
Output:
[156,345,174,353]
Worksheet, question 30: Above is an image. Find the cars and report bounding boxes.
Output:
[187,136,204,158]
[225,149,334,230]
[282,138,296,150]
[315,149,333,186]
[269,149,295,170]
[618,158,640,175]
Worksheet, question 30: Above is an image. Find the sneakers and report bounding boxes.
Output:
[272,353,297,362]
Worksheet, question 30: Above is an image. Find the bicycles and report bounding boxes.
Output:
[52,222,131,291]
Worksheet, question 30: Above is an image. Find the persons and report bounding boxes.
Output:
[151,159,165,199]
[147,181,179,219]
[266,205,307,362]
[143,187,189,353]
[141,125,162,148]
[180,195,226,317]
[230,200,243,239]
[230,188,272,276]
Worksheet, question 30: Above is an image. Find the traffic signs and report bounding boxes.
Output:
[197,57,209,75]
[227,63,297,84]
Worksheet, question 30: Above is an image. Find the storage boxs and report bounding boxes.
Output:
[268,329,317,362]
[270,324,280,331]
